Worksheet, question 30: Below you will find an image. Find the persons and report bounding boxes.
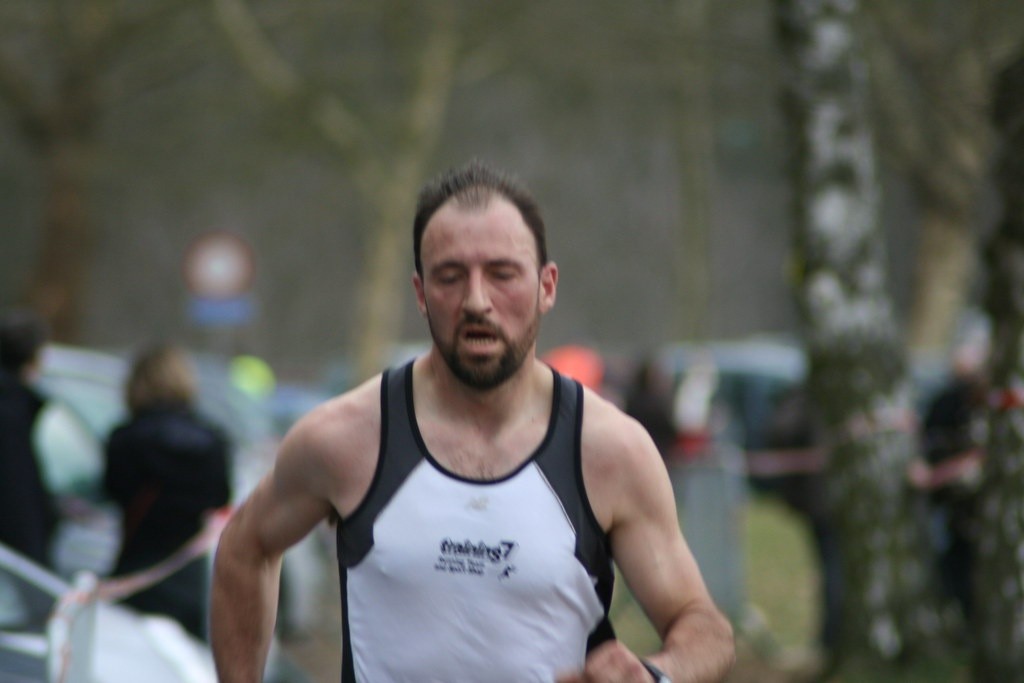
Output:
[210,158,733,683]
[99,347,235,640]
[0,320,101,568]
[625,362,686,460]
[908,346,991,596]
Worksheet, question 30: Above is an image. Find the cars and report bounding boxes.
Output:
[40,344,341,641]
[680,330,820,507]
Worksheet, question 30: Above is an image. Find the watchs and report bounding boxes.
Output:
[640,658,671,683]
